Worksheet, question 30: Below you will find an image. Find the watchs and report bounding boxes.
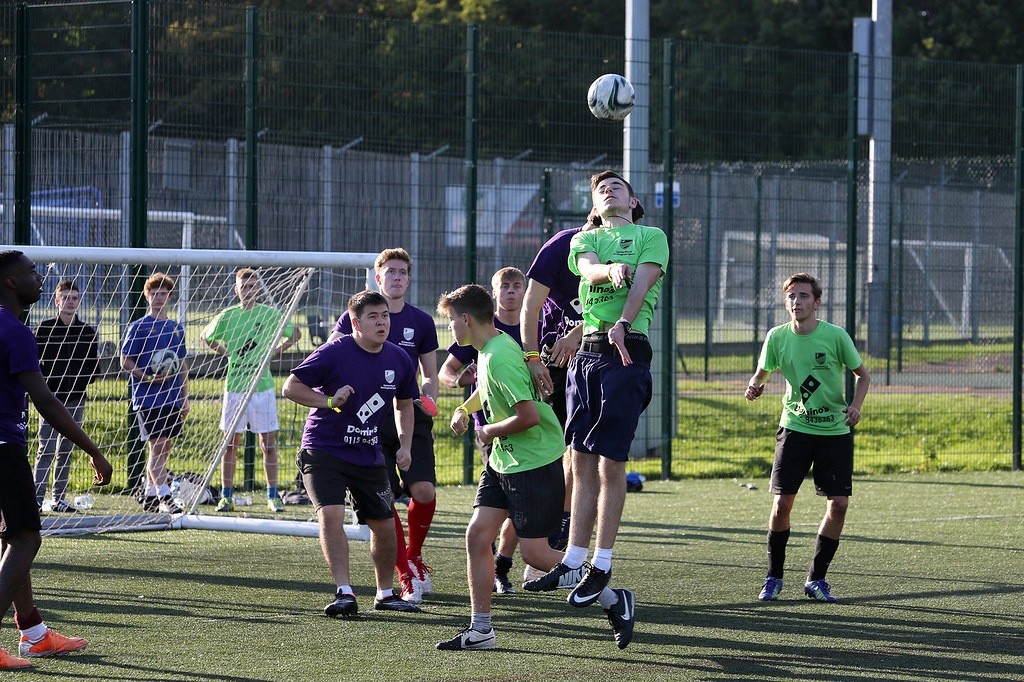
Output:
[617,320,631,333]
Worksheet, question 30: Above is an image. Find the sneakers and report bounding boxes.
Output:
[160,494,183,514]
[267,497,286,511]
[524,566,548,581]
[567,563,613,608]
[758,576,784,601]
[37,502,44,513]
[548,516,571,551]
[323,588,358,617]
[143,496,159,512]
[604,587,635,649]
[406,546,434,595]
[493,570,516,594]
[19,628,90,657]
[214,497,235,512]
[373,588,421,613]
[0,647,33,669]
[397,572,424,605]
[804,576,838,603]
[521,561,590,592]
[435,622,496,650]
[51,500,81,513]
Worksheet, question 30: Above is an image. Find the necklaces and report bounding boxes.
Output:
[605,215,632,224]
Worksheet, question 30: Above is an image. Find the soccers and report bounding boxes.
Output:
[587,73,635,121]
[151,349,180,375]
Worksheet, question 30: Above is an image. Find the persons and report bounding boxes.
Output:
[748,273,870,604]
[32,280,99,514]
[282,291,419,615]
[438,199,642,594]
[328,248,439,601]
[434,285,635,649]
[201,269,303,511]
[121,273,190,513]
[0,252,114,669]
[523,169,669,606]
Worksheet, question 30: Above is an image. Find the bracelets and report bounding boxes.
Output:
[608,267,612,280]
[525,351,539,356]
[455,379,460,387]
[526,356,539,360]
[454,407,468,415]
[147,376,151,384]
[328,397,341,413]
